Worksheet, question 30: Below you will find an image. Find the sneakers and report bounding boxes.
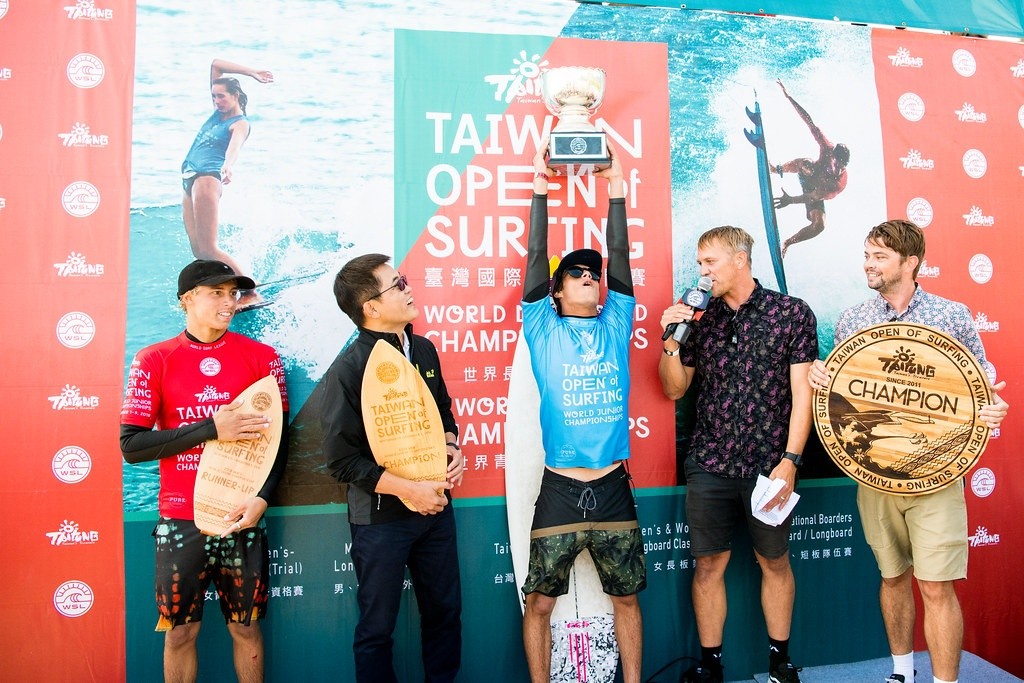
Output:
[683,665,724,683]
[768,657,802,683]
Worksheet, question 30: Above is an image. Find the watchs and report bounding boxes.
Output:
[779,452,805,468]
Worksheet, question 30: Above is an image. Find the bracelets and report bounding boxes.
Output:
[444,442,462,450]
[663,349,682,358]
[533,172,550,180]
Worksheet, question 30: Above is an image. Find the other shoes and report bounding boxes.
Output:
[768,161,781,174]
[782,239,789,259]
[885,669,917,683]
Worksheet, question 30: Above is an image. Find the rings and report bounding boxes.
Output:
[781,497,785,501]
[235,523,241,529]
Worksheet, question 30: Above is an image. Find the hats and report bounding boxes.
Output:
[552,249,602,307]
[833,144,849,166]
[177,260,255,300]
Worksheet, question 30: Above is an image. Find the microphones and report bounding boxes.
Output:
[661,277,714,341]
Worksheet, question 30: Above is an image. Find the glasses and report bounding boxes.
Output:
[361,275,407,310]
[562,266,600,280]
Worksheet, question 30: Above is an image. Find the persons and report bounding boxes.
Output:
[323,253,466,683]
[522,139,649,683]
[808,221,1009,683]
[118,259,290,683]
[179,58,275,311]
[767,77,850,257]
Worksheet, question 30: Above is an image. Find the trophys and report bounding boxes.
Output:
[540,66,614,177]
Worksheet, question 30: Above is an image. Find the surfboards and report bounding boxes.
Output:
[230,300,279,315]
[505,312,620,683]
[743,84,791,297]
